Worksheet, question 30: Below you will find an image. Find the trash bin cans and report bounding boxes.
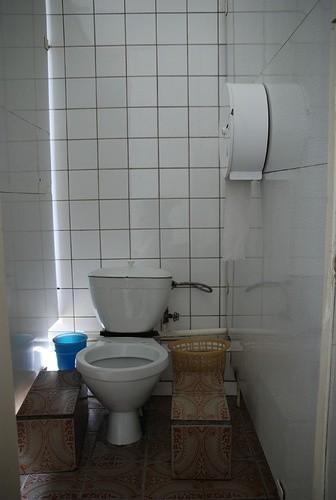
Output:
[169,336,231,382]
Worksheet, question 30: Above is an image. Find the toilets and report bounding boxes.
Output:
[74,259,173,446]
